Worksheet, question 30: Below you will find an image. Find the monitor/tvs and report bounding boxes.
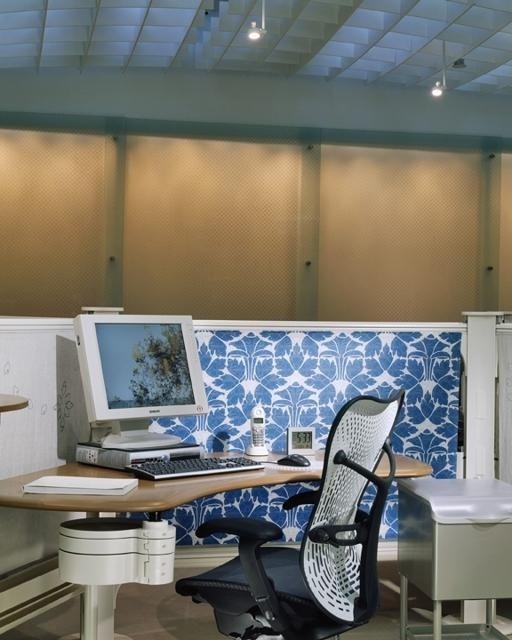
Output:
[73,313,209,451]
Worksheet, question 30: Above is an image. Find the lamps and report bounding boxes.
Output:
[430,39,448,97]
[246,0,268,42]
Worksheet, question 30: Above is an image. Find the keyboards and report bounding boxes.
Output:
[124,456,265,480]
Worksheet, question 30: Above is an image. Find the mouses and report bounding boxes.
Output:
[278,454,310,467]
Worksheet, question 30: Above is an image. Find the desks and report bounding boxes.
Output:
[0,393,33,412]
[0,449,435,640]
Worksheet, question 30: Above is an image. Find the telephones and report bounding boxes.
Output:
[245,398,269,456]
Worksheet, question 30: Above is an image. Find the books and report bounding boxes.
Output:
[18,473,141,498]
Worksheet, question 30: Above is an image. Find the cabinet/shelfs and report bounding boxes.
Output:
[395,475,511,640]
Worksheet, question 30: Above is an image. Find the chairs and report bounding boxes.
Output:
[175,387,407,640]
